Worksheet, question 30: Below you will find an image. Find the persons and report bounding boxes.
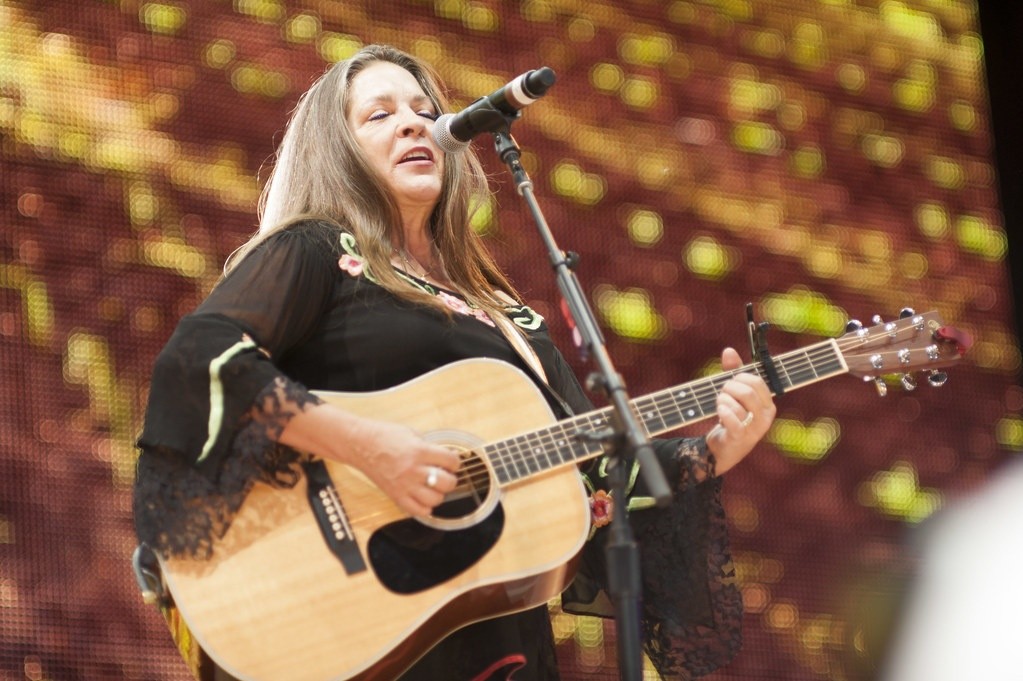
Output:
[130,46,778,681]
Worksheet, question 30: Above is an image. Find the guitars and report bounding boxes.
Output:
[138,307,966,681]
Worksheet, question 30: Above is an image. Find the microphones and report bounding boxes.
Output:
[432,67,556,154]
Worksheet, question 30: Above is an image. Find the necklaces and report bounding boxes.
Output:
[395,248,442,281]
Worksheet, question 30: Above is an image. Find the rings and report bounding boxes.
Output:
[427,468,438,487]
[743,412,753,424]
